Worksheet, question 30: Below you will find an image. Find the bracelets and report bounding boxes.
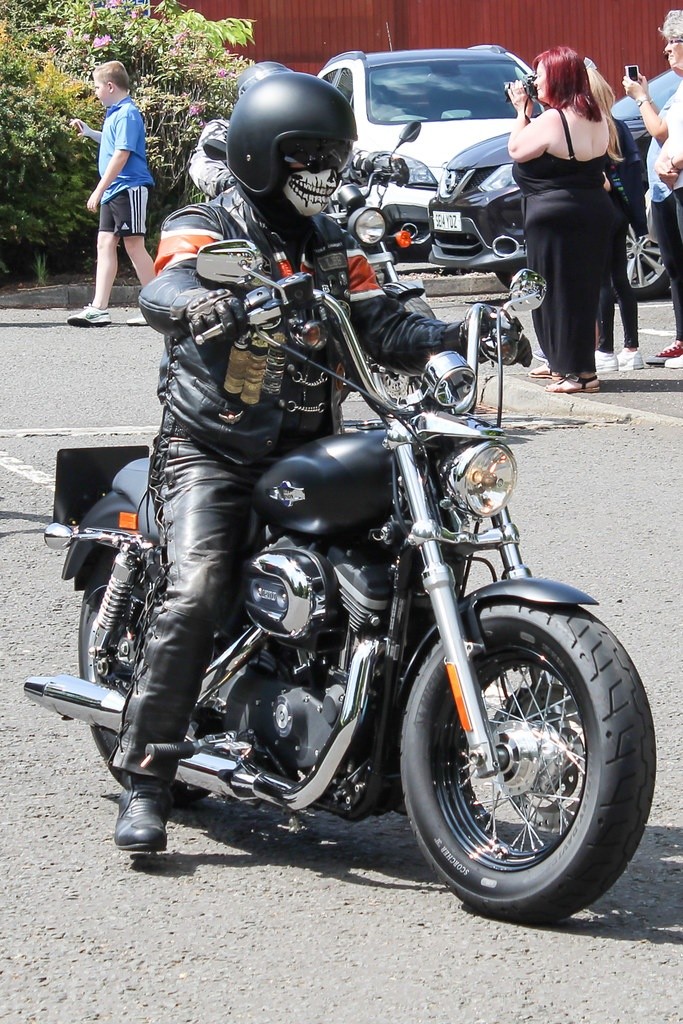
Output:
[650,98,653,104]
[670,156,682,171]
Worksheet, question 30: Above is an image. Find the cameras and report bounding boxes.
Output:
[504,76,538,103]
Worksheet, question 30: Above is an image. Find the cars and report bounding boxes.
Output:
[429,64,683,303]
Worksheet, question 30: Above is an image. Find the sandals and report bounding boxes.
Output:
[528,363,562,380]
[544,372,601,393]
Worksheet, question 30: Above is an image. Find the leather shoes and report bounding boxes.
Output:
[114,768,174,853]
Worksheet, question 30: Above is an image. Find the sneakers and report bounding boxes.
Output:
[664,352,683,369]
[66,302,112,327]
[595,349,619,372]
[126,311,148,326]
[615,347,643,371]
[646,341,683,364]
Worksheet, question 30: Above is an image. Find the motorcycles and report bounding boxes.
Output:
[21,245,657,924]
[203,121,438,417]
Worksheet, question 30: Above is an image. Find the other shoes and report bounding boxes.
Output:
[530,346,548,363]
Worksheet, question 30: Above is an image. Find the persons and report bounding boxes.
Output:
[66,59,156,326]
[584,67,648,373]
[622,9,683,368]
[507,46,615,393]
[107,73,532,852]
[189,61,410,200]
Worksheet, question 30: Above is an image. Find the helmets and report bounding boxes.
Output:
[237,61,294,100]
[226,72,358,194]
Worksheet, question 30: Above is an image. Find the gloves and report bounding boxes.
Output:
[169,286,248,344]
[459,306,533,367]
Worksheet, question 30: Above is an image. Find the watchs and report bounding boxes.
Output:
[636,98,647,106]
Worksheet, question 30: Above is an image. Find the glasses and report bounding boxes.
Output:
[664,36,683,47]
[278,138,351,176]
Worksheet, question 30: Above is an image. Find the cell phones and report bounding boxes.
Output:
[625,65,639,84]
[75,122,83,133]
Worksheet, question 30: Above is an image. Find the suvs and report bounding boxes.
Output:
[317,48,544,264]
